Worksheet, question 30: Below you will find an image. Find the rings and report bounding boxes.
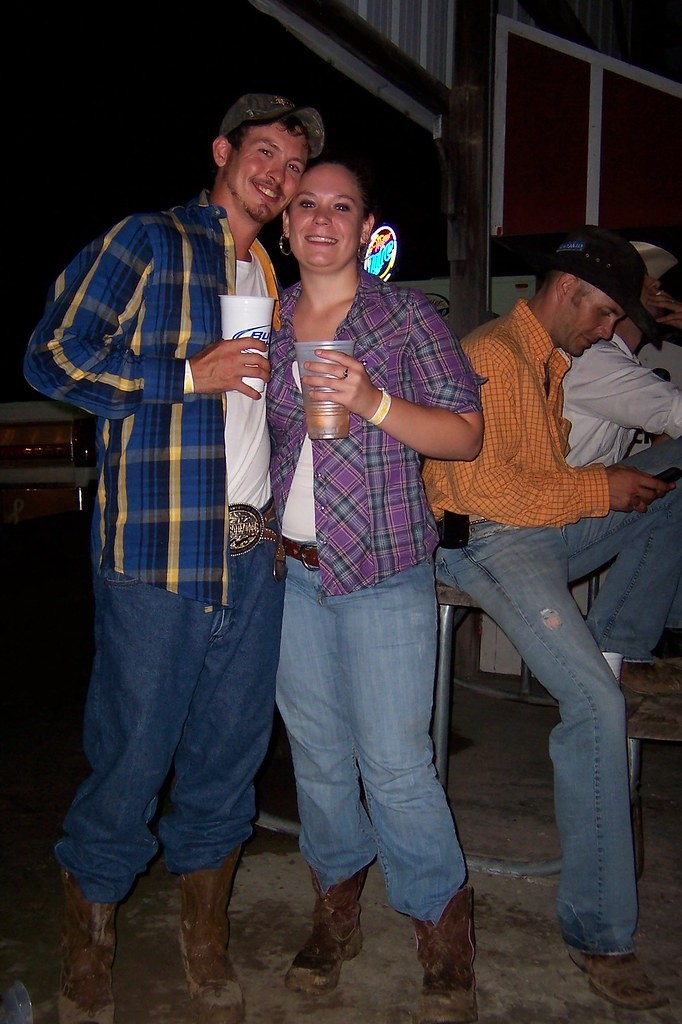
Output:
[344,368,348,378]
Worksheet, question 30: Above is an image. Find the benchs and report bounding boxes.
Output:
[253,571,640,881]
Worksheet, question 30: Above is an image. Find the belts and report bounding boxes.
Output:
[283,539,319,571]
[228,499,287,583]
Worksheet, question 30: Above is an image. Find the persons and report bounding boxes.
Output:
[422,223,682,1012]
[25,89,325,1024]
[266,158,487,1024]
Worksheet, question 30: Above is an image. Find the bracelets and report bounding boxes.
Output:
[367,386,392,425]
[184,358,194,395]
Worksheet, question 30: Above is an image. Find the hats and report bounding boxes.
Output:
[218,92,325,158]
[629,241,682,304]
[550,224,662,352]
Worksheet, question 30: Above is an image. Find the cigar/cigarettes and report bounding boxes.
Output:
[655,291,663,296]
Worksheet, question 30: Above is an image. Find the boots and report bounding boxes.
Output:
[57,869,116,1024]
[411,883,477,1024]
[181,844,245,1024]
[284,858,368,995]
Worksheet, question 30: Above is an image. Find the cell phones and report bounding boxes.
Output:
[651,468,681,487]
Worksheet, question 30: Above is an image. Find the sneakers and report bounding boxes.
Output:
[569,948,670,1010]
[619,658,682,697]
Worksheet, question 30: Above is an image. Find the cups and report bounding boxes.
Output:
[602,652,623,687]
[219,294,277,394]
[293,339,357,440]
